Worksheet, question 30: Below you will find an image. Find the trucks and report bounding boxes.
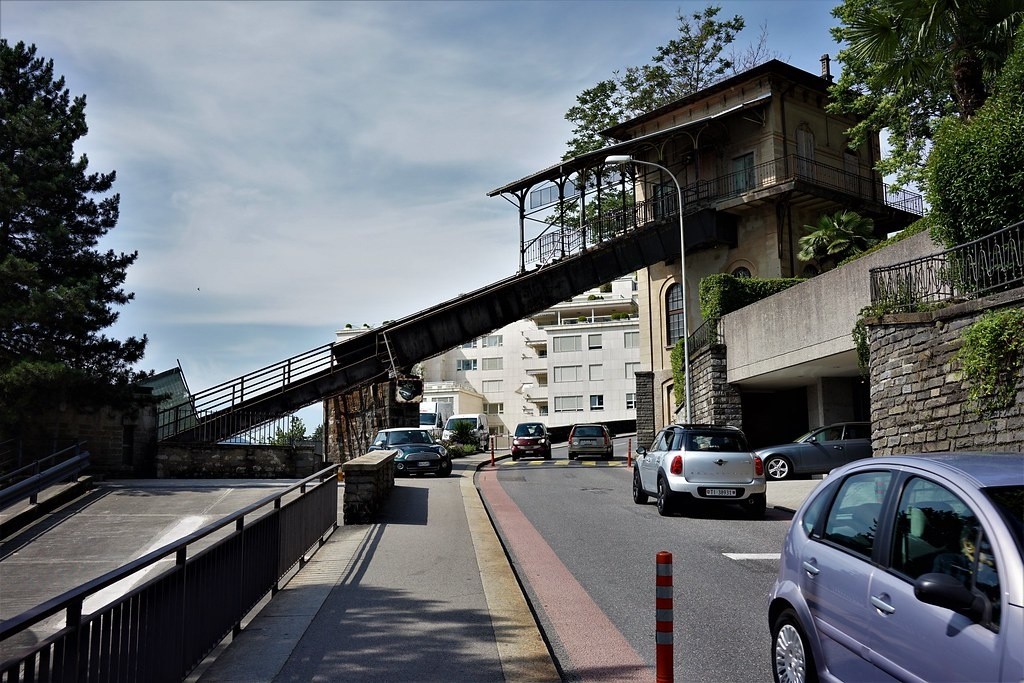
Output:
[419,401,454,442]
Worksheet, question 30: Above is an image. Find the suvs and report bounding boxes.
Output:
[632,423,768,520]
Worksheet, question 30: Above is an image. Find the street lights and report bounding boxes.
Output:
[604,155,691,425]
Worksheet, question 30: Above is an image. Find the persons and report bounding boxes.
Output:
[830,428,840,440]
[933,525,1001,610]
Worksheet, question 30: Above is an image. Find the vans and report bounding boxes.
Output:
[442,412,489,453]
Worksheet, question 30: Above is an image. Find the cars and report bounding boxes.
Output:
[767,451,1024,683]
[752,420,873,482]
[568,422,616,461]
[368,427,453,478]
[509,422,553,461]
